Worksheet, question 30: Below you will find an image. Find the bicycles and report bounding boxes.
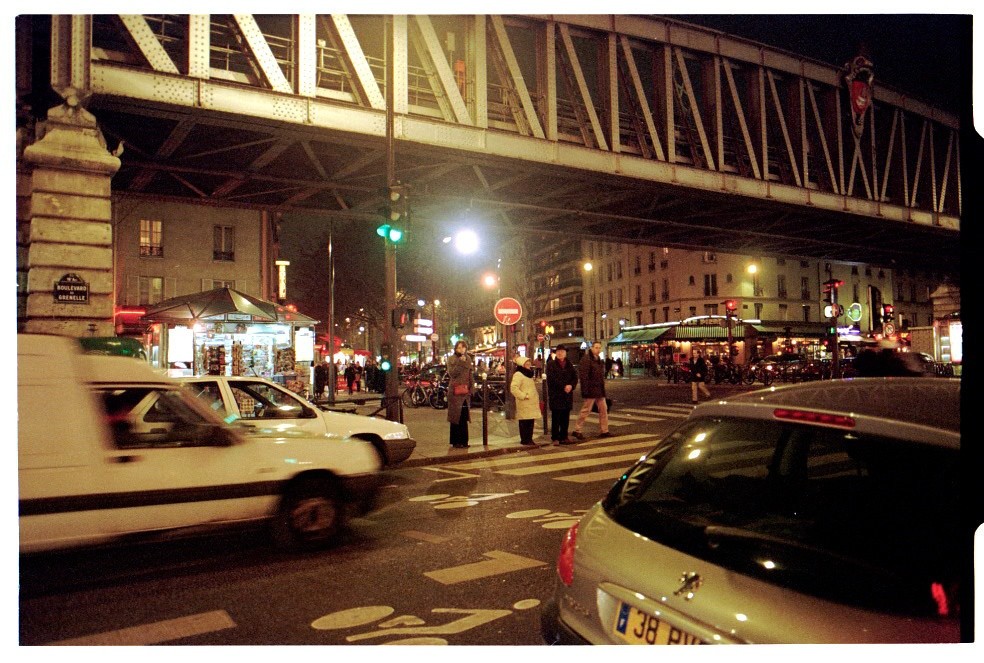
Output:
[664,364,854,386]
[398,368,482,411]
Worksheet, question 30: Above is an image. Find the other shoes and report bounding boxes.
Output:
[553,439,560,446]
[600,432,614,438]
[453,443,470,448]
[561,438,575,444]
[572,431,585,439]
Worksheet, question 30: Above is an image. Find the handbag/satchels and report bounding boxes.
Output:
[591,398,612,414]
[454,384,469,396]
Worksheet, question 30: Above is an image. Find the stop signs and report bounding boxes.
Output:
[494,297,523,326]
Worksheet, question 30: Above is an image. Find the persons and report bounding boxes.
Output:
[604,349,711,404]
[446,341,473,448]
[933,362,953,378]
[546,344,578,446]
[510,358,542,448]
[311,352,555,402]
[847,338,930,377]
[571,341,615,439]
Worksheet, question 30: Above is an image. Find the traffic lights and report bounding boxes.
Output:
[392,306,416,328]
[823,280,835,305]
[882,303,895,323]
[726,299,738,318]
[377,183,412,246]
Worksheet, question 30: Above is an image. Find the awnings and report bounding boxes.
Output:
[608,327,673,346]
[675,326,744,338]
[751,325,829,334]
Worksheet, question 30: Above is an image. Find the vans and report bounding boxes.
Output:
[750,354,808,375]
[18,334,384,557]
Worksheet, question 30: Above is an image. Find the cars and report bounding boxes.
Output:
[105,375,418,469]
[539,376,962,644]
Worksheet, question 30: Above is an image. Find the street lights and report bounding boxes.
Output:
[748,265,757,319]
[583,261,597,342]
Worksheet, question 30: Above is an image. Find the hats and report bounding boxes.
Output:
[552,344,569,353]
[515,357,531,367]
